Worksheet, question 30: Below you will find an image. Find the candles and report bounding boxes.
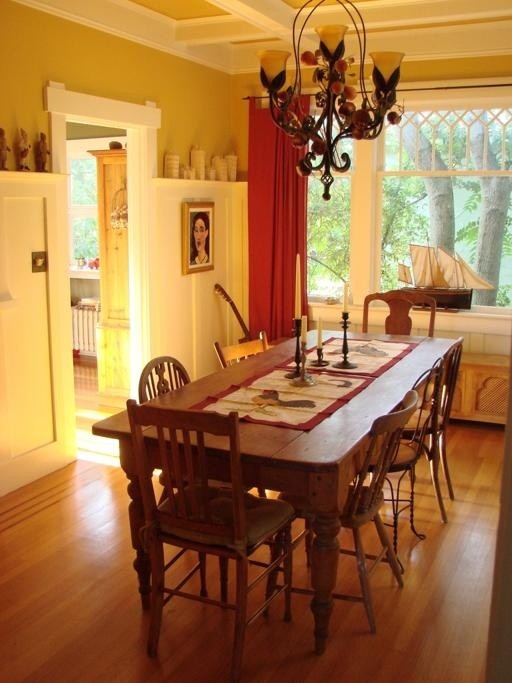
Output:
[343,282,349,314]
[301,315,307,342]
[317,316,322,348]
[294,253,302,320]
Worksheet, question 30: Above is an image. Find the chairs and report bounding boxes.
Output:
[261,390,419,634]
[352,356,446,575]
[401,336,464,524]
[212,330,269,369]
[126,399,297,683]
[138,356,274,602]
[362,289,437,337]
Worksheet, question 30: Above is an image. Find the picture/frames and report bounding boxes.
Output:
[182,200,215,276]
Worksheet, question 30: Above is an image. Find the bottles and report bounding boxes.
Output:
[164,146,238,181]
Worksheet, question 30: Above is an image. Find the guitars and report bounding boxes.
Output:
[215,283,253,342]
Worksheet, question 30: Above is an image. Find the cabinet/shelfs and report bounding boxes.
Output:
[86,149,134,410]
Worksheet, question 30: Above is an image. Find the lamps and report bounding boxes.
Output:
[256,0,405,200]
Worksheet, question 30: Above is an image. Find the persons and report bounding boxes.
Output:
[37,132,50,172]
[0,128,11,170]
[189,212,209,265]
[19,128,32,170]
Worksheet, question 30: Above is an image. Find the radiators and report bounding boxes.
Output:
[72,304,101,357]
